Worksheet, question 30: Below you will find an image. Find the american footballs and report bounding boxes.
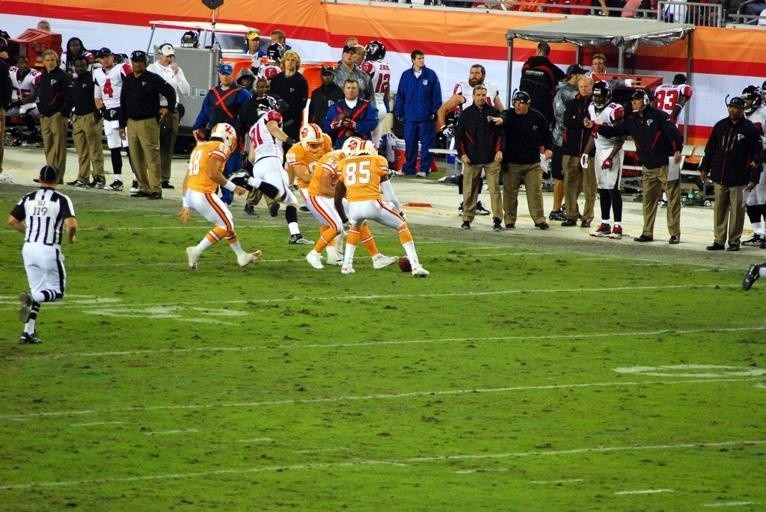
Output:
[398,257,413,273]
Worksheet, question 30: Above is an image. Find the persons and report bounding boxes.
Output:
[653,73,694,208]
[732,85,766,249]
[337,139,429,276]
[308,136,363,270]
[7,164,75,347]
[761,80,766,103]
[742,263,766,291]
[286,124,343,268]
[698,96,763,252]
[179,121,262,268]
[336,1,766,29]
[3,28,632,239]
[225,93,316,246]
[582,89,685,245]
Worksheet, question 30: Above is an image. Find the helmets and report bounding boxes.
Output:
[211,96,374,155]
[445,111,461,128]
[365,40,386,60]
[514,64,761,115]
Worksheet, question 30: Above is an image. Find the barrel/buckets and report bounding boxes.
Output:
[392,145,421,174]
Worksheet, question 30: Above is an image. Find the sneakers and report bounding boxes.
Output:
[18,291,32,323]
[396,168,405,175]
[18,332,43,344]
[416,168,431,177]
[185,236,430,277]
[67,169,280,218]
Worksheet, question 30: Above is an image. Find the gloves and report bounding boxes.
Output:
[579,153,589,169]
[441,125,454,137]
[601,157,613,169]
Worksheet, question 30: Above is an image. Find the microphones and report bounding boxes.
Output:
[511,88,517,100]
[725,95,729,107]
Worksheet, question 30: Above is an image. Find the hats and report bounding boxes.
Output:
[40,165,60,179]
[94,34,356,75]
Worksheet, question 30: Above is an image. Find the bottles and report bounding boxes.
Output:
[679,184,702,207]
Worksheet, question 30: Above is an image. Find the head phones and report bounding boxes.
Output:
[512,91,532,106]
[158,43,172,54]
[244,31,259,46]
[635,89,650,105]
[142,51,150,66]
